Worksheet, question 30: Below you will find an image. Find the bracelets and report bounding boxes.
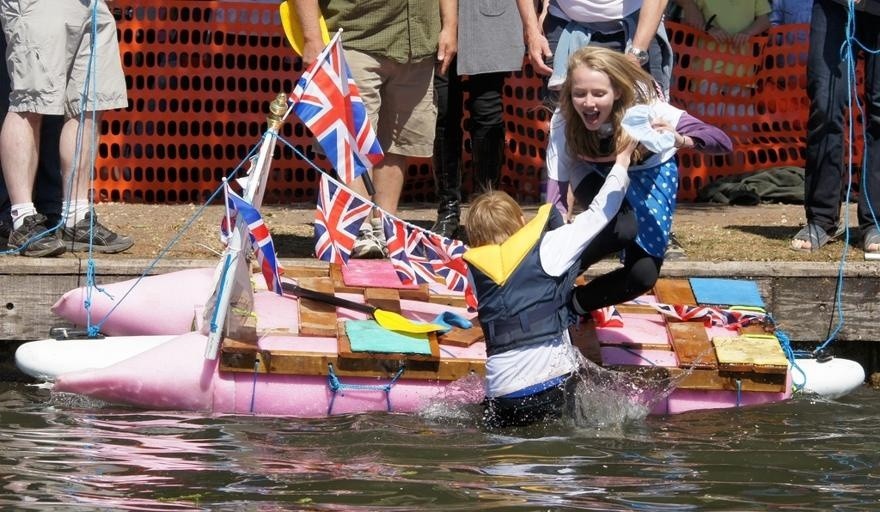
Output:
[674,132,687,149]
[705,14,716,35]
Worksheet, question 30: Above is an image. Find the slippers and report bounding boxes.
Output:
[864,228,880,254]
[790,221,845,253]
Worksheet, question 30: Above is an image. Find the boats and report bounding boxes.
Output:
[8,252,868,419]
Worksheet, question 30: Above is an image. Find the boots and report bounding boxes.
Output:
[469,123,505,202]
[431,128,463,239]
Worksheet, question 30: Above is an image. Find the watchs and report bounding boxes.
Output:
[627,48,650,67]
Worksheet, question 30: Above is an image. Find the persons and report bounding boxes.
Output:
[90,0,291,203]
[513,0,687,263]
[457,128,643,426]
[542,44,735,327]
[0,1,136,258]
[766,0,814,168]
[676,0,773,190]
[790,0,879,260]
[429,0,550,241]
[287,0,460,259]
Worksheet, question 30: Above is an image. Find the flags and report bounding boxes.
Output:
[420,230,478,311]
[377,209,425,290]
[222,182,286,300]
[289,31,387,188]
[591,302,778,332]
[310,173,374,270]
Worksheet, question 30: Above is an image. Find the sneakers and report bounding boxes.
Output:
[7,213,66,257]
[55,210,134,253]
[349,218,389,259]
